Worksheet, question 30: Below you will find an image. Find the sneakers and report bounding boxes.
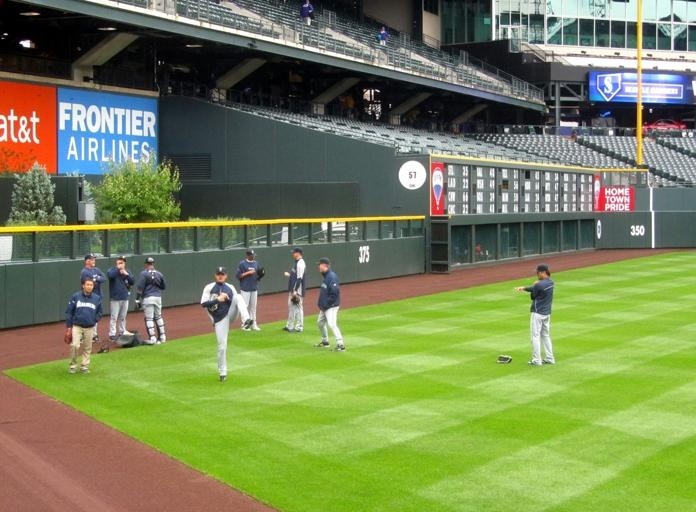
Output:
[244,319,254,329]
[330,344,346,353]
[70,367,90,375]
[220,375,227,382]
[528,359,552,366]
[282,327,301,332]
[314,342,330,348]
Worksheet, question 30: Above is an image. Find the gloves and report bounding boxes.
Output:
[135,299,141,309]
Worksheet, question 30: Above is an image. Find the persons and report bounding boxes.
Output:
[200,266,254,381]
[300,0,315,27]
[107,257,135,337]
[283,247,306,331]
[314,258,345,352]
[235,248,267,328]
[379,27,390,46]
[515,263,554,366]
[81,254,106,342]
[137,257,165,343]
[65,279,104,374]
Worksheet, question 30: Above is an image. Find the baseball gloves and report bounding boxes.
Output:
[496,355,512,363]
[64,328,73,345]
[292,295,300,304]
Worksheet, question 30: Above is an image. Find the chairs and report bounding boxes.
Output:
[208,104,695,185]
[124,0,544,107]
[521,42,696,71]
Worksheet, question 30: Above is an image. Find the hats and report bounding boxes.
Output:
[117,255,127,261]
[315,257,330,264]
[145,257,155,263]
[534,264,548,271]
[291,247,303,253]
[85,253,97,260]
[246,248,256,257]
[215,266,227,274]
